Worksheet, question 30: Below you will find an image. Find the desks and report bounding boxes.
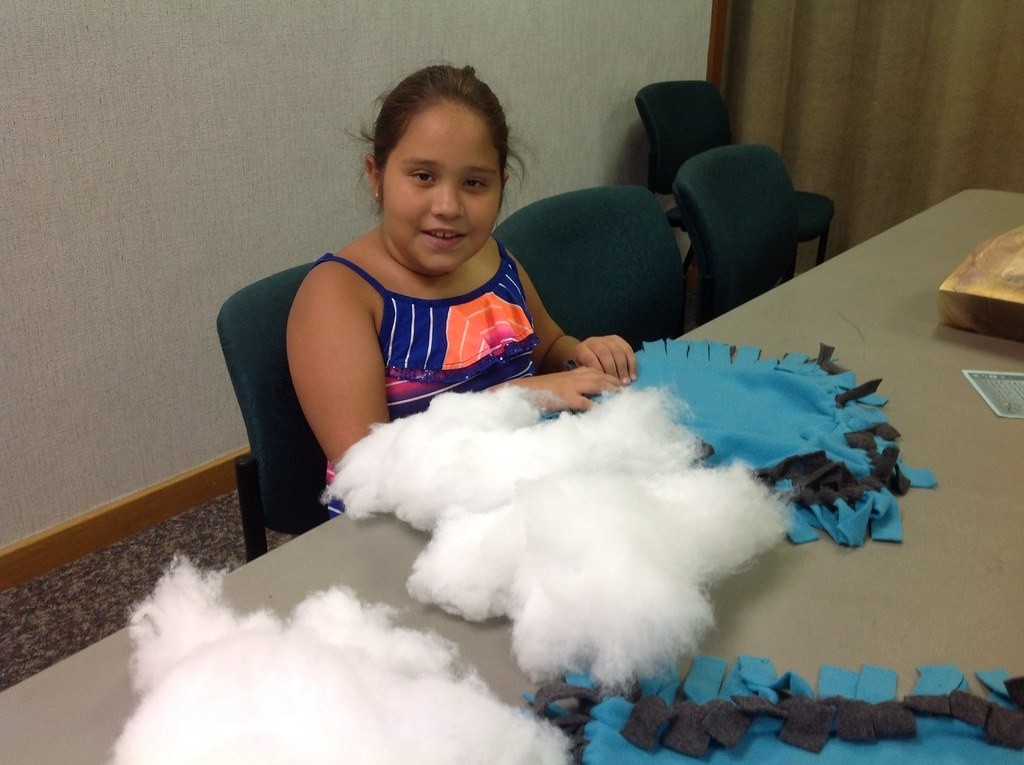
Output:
[1,189,1024,765]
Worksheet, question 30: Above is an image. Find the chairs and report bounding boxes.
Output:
[491,184,687,394]
[673,144,800,326]
[635,80,834,266]
[216,262,332,564]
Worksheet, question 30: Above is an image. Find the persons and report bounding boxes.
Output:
[287,64,636,521]
